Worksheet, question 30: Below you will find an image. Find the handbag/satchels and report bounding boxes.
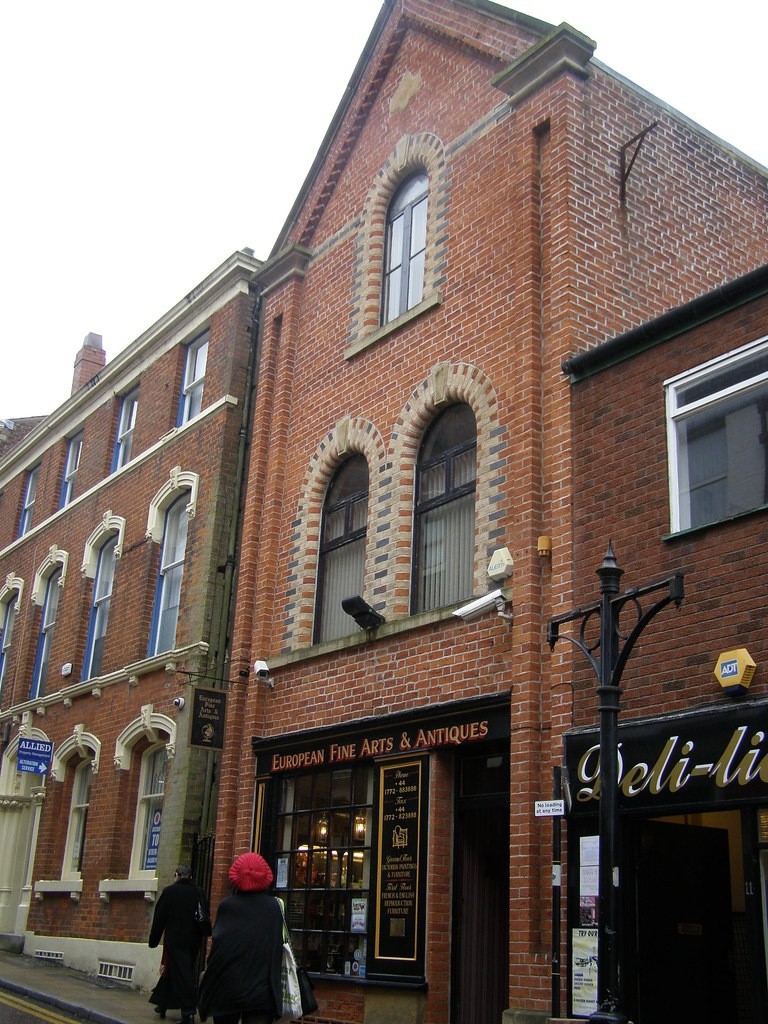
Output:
[194,887,213,938]
[276,897,318,1019]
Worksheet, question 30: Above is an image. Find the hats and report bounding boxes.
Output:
[230,853,274,891]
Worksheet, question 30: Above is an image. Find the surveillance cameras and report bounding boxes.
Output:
[174,698,185,710]
[253,660,270,681]
[450,589,506,622]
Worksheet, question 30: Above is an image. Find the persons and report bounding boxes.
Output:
[148,865,213,1024]
[196,852,293,1024]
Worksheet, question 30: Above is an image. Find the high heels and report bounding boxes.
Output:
[176,1014,193,1024]
[155,1005,167,1018]
